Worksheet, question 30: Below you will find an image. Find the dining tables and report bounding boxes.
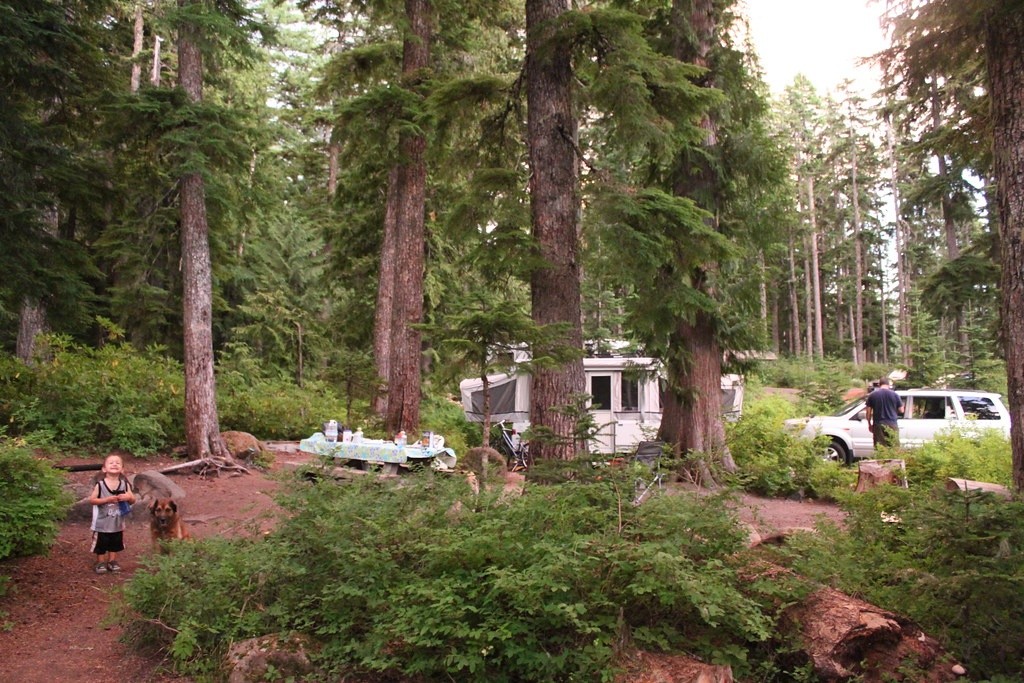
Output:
[301,433,448,479]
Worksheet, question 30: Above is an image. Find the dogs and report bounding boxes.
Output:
[149,498,191,554]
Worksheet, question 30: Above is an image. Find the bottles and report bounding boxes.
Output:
[396,433,402,447]
[342,426,352,442]
[401,430,408,445]
[325,420,338,443]
[353,428,364,443]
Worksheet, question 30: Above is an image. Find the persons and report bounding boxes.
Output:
[866,378,904,450]
[90,451,135,573]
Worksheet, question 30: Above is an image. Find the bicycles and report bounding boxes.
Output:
[491,419,530,468]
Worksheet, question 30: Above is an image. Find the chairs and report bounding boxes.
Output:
[615,440,668,510]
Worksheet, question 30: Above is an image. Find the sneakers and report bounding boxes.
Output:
[93,562,108,574]
[107,560,122,571]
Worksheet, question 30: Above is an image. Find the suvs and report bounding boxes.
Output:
[800,386,1011,468]
